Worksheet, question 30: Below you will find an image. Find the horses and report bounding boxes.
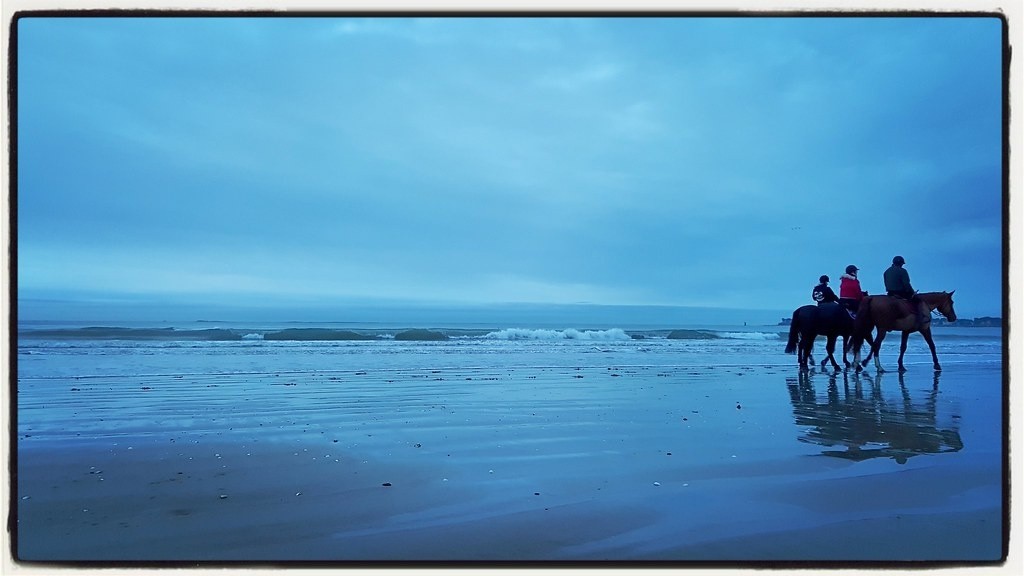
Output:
[785,290,956,370]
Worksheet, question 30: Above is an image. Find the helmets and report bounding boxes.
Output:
[819,275,829,282]
[846,265,859,274]
[893,256,905,264]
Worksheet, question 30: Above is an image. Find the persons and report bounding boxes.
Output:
[840,265,865,311]
[812,275,840,308]
[884,256,925,330]
